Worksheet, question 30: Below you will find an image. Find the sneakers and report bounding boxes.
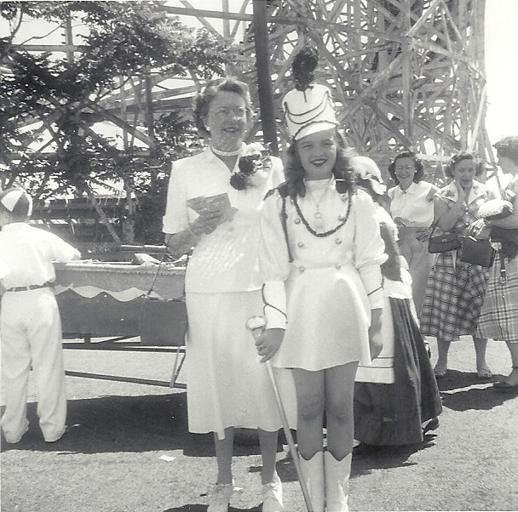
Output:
[257,474,286,512]
[204,478,236,512]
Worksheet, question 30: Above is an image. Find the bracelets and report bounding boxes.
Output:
[186,227,202,240]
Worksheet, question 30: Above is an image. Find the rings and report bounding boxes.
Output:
[259,346,263,351]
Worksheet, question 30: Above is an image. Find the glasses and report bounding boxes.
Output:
[202,106,250,121]
[393,165,415,172]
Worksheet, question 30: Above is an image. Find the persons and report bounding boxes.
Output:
[254,130,384,512]
[158,75,286,512]
[348,155,443,455]
[473,136,517,391]
[0,188,82,444]
[419,150,493,379]
[384,150,438,359]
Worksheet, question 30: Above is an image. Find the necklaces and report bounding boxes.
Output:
[212,145,242,157]
[306,181,333,220]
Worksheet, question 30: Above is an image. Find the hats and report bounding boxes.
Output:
[280,82,340,141]
[476,197,514,219]
[0,187,33,217]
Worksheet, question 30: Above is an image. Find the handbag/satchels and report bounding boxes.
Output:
[425,216,461,253]
[458,233,497,268]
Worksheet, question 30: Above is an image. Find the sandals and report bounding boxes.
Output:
[430,363,448,377]
[491,366,518,392]
[475,366,493,379]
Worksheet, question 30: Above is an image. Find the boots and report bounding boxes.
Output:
[295,448,354,512]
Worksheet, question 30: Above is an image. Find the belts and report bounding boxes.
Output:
[5,280,51,294]
